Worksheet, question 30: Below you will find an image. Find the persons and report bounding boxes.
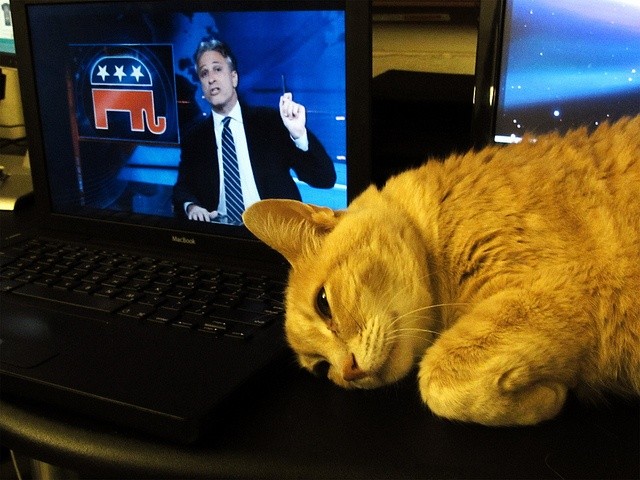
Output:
[171,35,336,223]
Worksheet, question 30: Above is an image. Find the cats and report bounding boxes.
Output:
[240,111,640,427]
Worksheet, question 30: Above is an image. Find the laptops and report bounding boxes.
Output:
[0,0,374,479]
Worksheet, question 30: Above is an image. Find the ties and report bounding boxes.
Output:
[222,117,245,226]
